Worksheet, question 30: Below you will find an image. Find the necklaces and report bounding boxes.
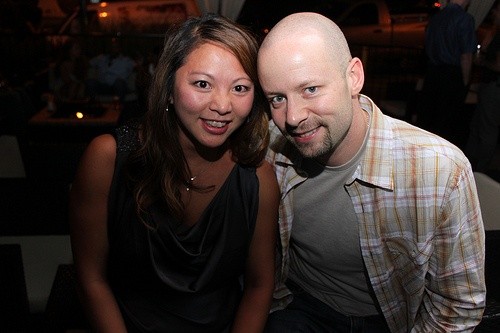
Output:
[186,176,198,192]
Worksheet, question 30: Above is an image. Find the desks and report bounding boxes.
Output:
[30,103,122,125]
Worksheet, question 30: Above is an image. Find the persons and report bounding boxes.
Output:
[255,12,487,333]
[67,12,281,332]
[0,0,500,172]
